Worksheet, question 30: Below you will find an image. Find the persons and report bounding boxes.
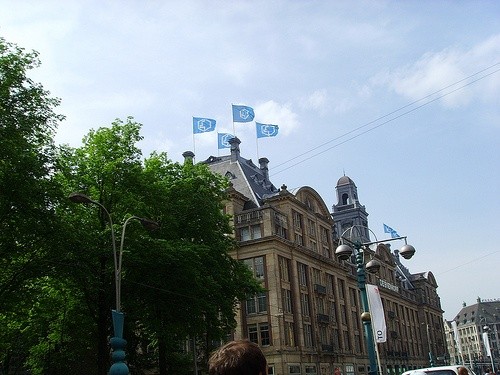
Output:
[335,367,340,375]
[209,339,268,375]
[492,369,500,375]
[458,367,469,375]
[485,368,490,375]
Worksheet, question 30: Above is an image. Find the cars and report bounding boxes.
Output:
[402,365,476,375]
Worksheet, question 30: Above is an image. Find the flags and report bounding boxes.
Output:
[218,133,235,149]
[256,122,279,138]
[451,321,462,353]
[391,232,400,238]
[483,332,491,356]
[384,224,397,233]
[366,284,386,343]
[193,116,216,134]
[232,105,255,122]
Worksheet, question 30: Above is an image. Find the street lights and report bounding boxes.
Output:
[482,325,496,374]
[333,225,417,375]
[68,192,160,375]
